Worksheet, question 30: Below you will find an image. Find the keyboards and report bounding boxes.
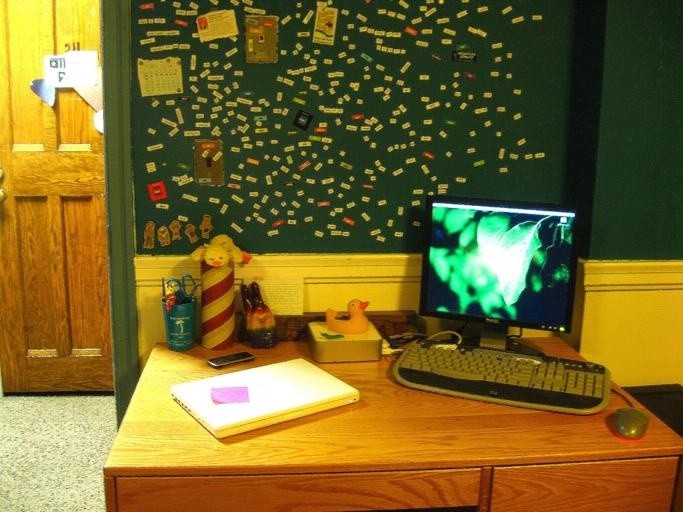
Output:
[393,340,611,415]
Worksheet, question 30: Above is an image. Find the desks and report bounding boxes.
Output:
[104,336,683,512]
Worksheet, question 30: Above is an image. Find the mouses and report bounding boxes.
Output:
[614,408,649,440]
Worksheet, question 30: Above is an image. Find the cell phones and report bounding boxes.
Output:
[208,352,255,369]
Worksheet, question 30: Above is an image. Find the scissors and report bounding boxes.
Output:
[166,274,197,298]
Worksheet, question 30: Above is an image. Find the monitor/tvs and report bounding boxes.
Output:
[419,195,579,356]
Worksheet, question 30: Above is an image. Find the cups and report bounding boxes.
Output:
[161,296,196,351]
